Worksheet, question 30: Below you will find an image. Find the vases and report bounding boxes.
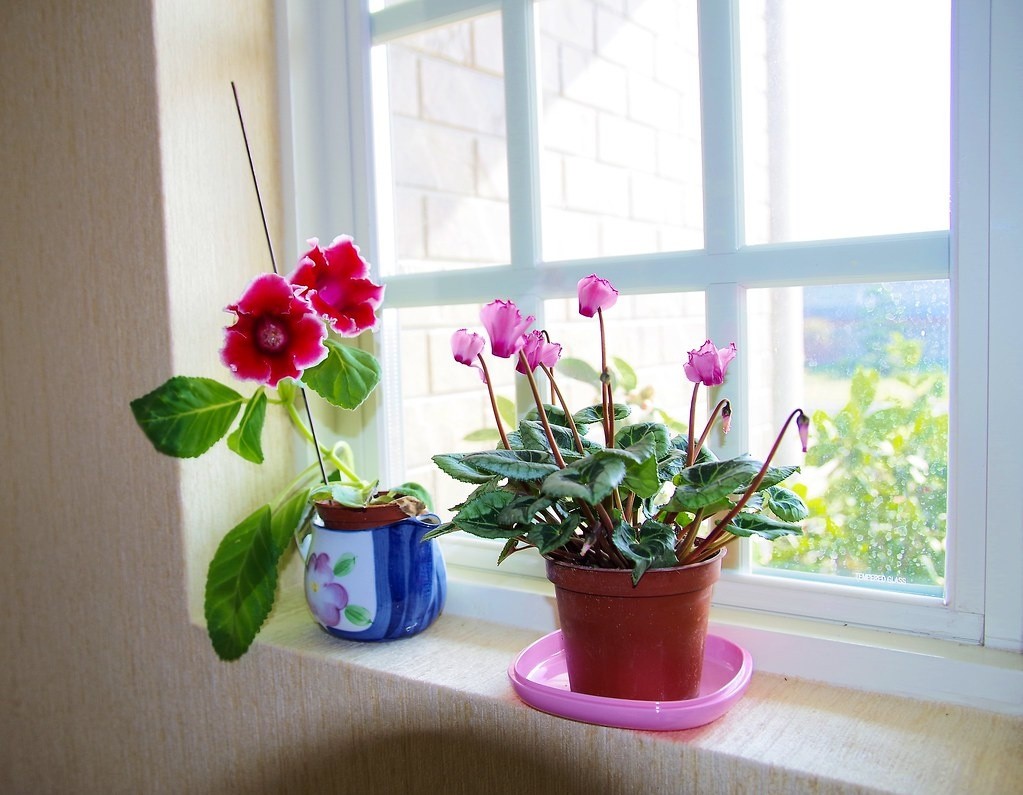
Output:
[304,493,446,641]
[505,527,754,729]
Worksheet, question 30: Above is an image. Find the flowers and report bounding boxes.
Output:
[130,234,439,663]
[420,274,811,587]
